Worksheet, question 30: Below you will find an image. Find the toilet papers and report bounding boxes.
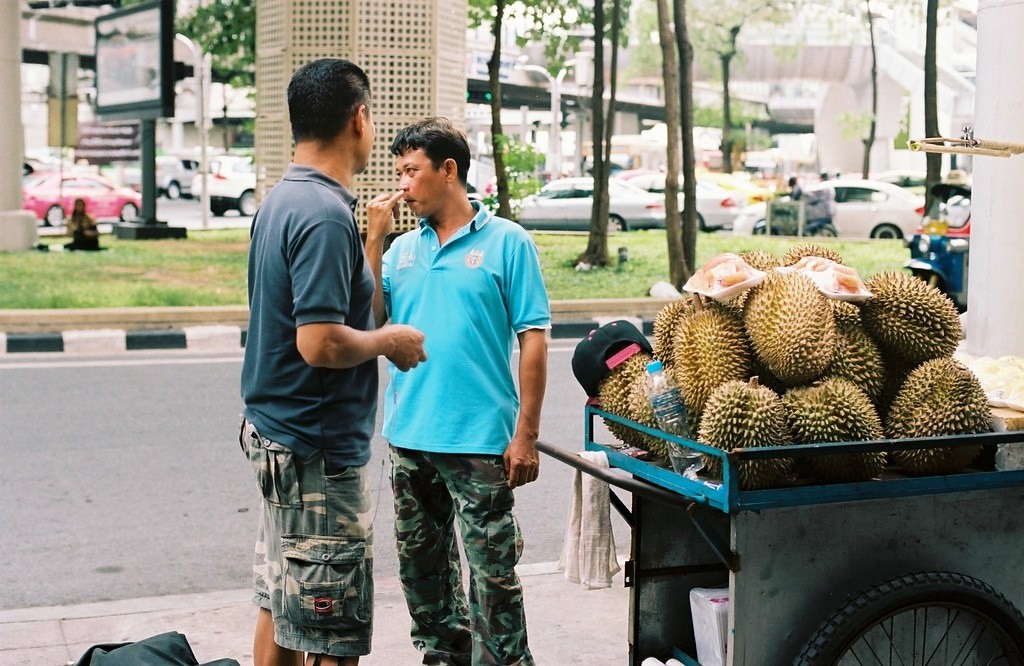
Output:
[665,659,686,666]
[641,657,666,666]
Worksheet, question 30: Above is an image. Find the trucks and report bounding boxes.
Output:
[123,154,210,199]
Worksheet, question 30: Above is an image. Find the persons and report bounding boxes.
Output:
[237,59,428,666]
[788,177,802,200]
[365,119,552,666]
[809,172,836,220]
[67,199,96,236]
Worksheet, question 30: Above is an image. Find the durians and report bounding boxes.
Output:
[597,244,1024,487]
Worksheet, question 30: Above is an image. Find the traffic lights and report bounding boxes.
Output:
[466,90,491,103]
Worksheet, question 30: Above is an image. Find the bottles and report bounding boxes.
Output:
[645,360,705,475]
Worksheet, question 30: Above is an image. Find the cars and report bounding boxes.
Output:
[734,178,926,239]
[191,155,256,216]
[488,169,770,234]
[23,151,142,226]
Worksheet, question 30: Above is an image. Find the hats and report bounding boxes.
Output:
[572,320,654,397]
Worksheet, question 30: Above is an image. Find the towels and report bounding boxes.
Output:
[557,450,622,591]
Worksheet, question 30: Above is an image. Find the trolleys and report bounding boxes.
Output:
[533,403,1024,666]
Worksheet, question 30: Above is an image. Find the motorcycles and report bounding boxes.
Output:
[903,171,970,312]
[753,195,838,236]
